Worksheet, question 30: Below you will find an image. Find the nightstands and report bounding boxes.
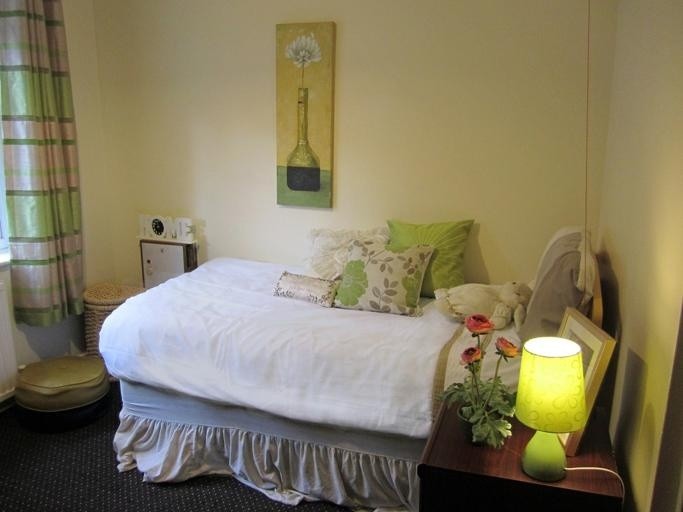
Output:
[417,402,628,511]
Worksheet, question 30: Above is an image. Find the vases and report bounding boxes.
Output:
[458,404,504,446]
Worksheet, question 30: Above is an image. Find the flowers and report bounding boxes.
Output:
[442,310,520,449]
[281,32,323,89]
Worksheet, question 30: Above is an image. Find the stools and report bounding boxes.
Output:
[13,354,110,432]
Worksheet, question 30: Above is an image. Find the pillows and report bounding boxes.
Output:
[383,220,475,298]
[521,223,593,353]
[302,227,381,276]
[271,270,340,307]
[333,239,433,318]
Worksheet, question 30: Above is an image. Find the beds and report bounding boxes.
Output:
[91,227,605,511]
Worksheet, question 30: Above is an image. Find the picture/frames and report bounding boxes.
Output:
[555,307,615,458]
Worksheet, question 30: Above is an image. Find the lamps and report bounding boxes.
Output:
[512,336,628,497]
